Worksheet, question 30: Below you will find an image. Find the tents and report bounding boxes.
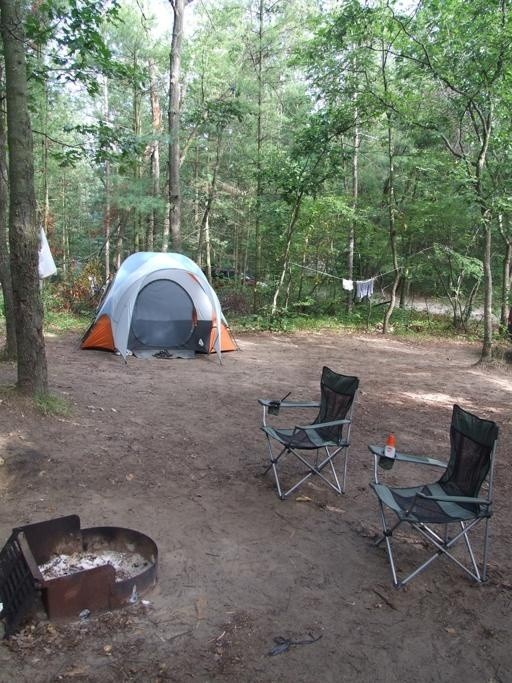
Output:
[78,252,243,366]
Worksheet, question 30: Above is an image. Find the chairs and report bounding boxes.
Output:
[259,365,360,501]
[367,404,498,588]
